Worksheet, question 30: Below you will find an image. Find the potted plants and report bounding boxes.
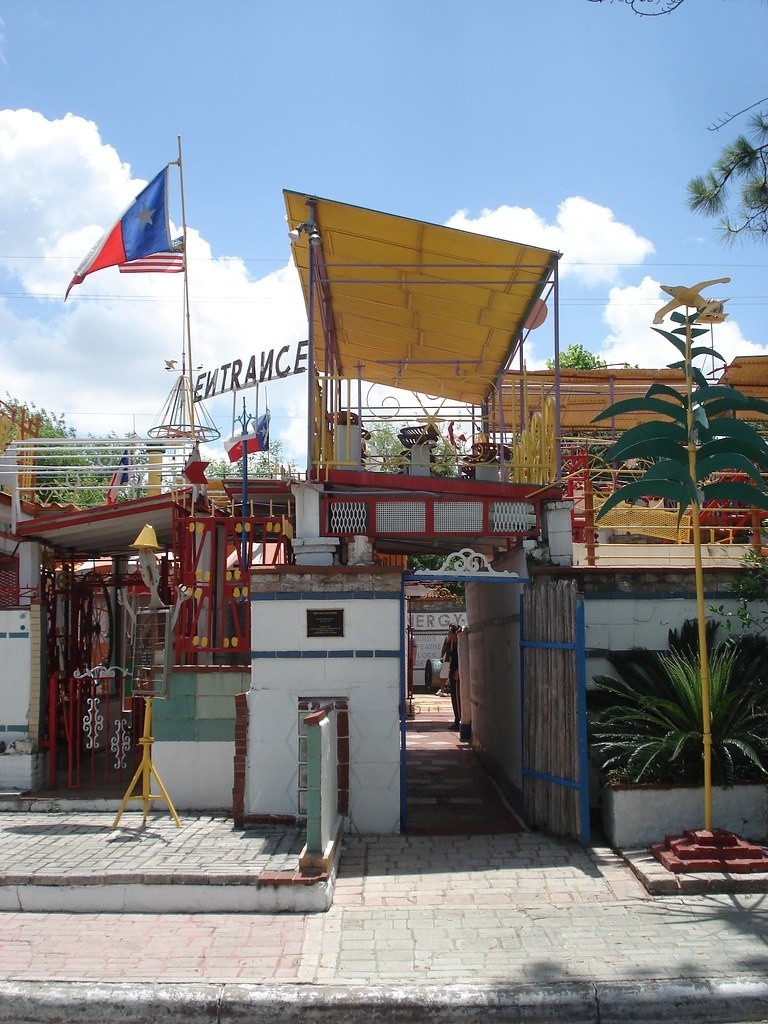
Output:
[595,617,768,847]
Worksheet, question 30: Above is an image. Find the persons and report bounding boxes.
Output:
[408,634,416,691]
[435,622,460,731]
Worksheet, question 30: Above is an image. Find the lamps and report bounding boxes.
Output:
[309,227,323,247]
[288,224,305,244]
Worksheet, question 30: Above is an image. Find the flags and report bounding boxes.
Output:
[222,412,270,462]
[65,165,175,300]
[118,235,185,273]
[107,449,129,504]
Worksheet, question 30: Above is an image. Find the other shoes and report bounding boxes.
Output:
[436,691,443,696]
[448,723,460,731]
